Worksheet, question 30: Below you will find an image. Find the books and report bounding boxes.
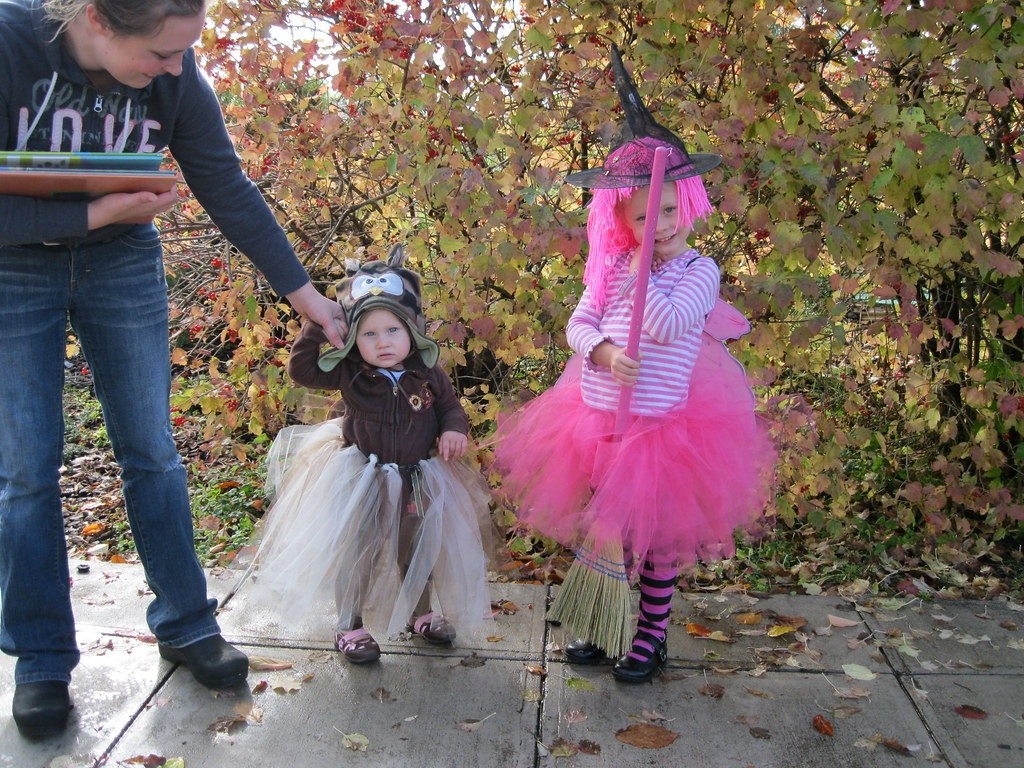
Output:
[0,151,177,197]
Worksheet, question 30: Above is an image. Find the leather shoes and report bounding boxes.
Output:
[159,631,250,690]
[565,633,605,662]
[11,678,72,739]
[612,627,668,680]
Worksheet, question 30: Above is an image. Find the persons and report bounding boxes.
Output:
[0,1,347,743]
[219,245,500,665]
[479,39,781,684]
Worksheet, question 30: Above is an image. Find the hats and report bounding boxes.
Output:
[316,244,440,374]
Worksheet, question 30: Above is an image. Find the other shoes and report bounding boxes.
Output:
[335,626,380,662]
[404,612,456,643]
